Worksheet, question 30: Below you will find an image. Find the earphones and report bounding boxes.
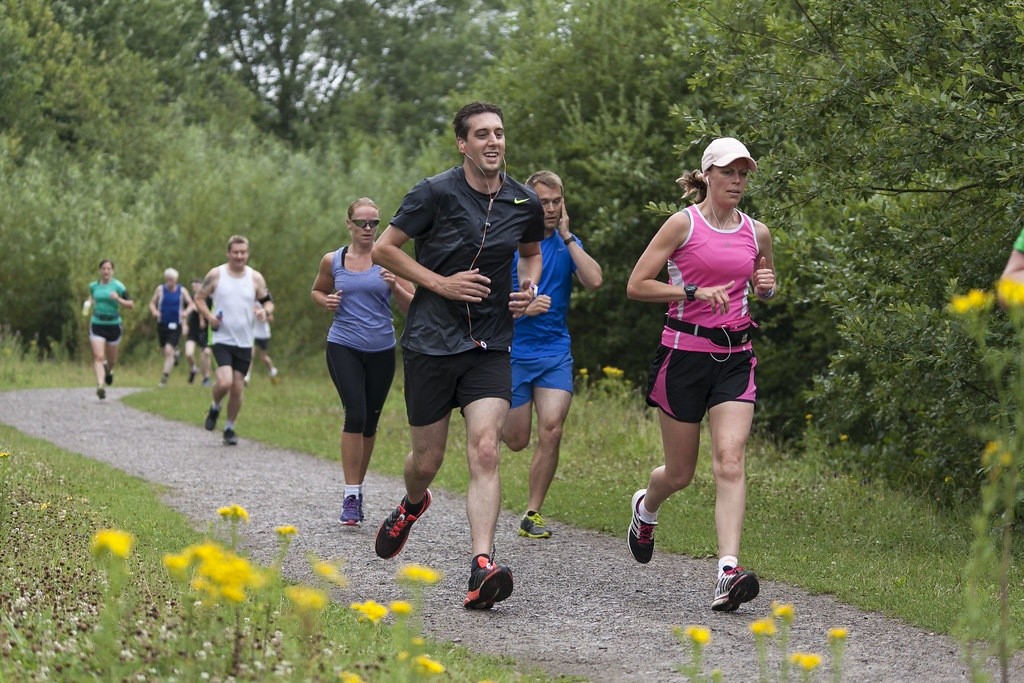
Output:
[705,175,710,187]
[461,144,467,152]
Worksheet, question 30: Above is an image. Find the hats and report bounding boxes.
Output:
[699,138,758,175]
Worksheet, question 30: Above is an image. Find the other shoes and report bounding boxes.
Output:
[268,368,279,377]
[160,372,170,384]
[175,351,181,366]
[98,387,106,399]
[202,377,211,386]
[223,428,237,446]
[188,371,197,382]
[243,371,251,382]
[204,406,220,432]
[104,361,114,386]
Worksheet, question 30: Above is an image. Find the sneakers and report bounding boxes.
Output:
[518,511,552,539]
[627,490,659,564]
[710,564,760,612]
[374,487,432,560]
[462,543,513,611]
[341,491,364,525]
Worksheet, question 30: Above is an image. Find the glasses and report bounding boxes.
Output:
[349,219,382,231]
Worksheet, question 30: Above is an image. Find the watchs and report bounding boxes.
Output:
[528,283,538,299]
[564,234,576,245]
[684,283,698,302]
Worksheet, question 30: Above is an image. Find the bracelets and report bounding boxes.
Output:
[757,287,774,299]
[265,308,269,316]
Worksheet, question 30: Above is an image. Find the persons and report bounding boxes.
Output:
[626,136,778,613]
[999,228,1024,309]
[150,267,213,387]
[310,197,416,526]
[195,234,275,447]
[497,170,602,540]
[372,104,544,613]
[81,261,135,398]
